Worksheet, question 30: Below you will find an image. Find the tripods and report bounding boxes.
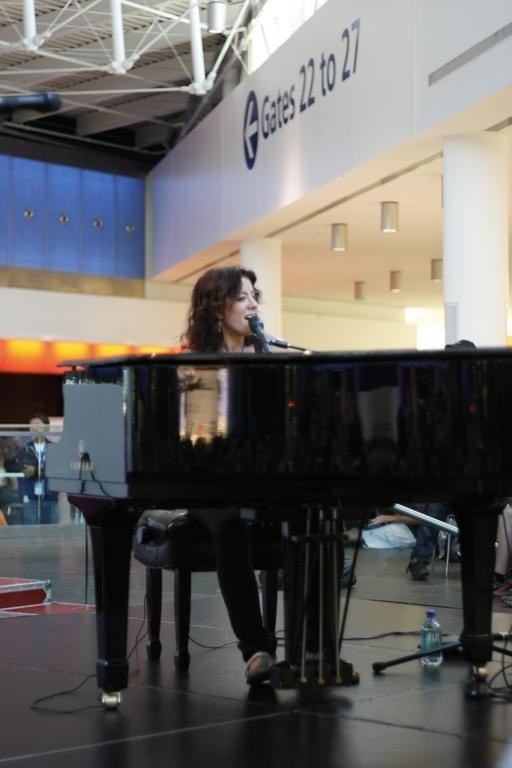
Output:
[374,631,512,674]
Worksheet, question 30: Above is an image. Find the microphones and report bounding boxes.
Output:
[249,316,272,353]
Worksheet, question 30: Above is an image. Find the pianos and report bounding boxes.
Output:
[45,347,510,708]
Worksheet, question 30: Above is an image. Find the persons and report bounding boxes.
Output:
[3,412,62,524]
[172,263,357,692]
[405,338,502,592]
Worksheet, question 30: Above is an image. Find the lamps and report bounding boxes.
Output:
[331,201,442,301]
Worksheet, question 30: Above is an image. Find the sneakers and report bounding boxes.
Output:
[492,574,512,604]
[407,561,430,579]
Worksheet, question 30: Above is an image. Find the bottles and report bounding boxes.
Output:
[420,610,443,667]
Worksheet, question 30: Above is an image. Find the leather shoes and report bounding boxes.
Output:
[244,652,272,684]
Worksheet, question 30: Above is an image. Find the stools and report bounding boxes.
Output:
[137,531,289,672]
